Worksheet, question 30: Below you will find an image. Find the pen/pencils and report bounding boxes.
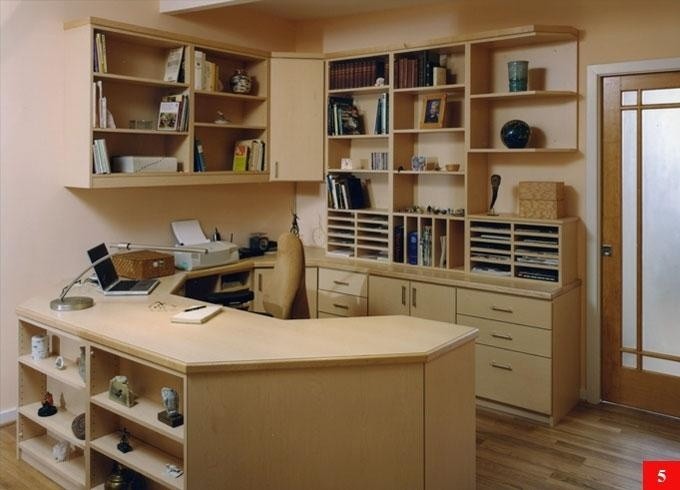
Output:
[185,306,206,311]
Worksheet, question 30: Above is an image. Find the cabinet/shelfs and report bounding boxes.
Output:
[457,281,583,427]
[18,308,187,488]
[317,265,368,320]
[468,213,580,287]
[255,264,318,320]
[468,25,583,154]
[368,274,457,324]
[270,53,327,184]
[59,17,268,191]
[327,38,468,274]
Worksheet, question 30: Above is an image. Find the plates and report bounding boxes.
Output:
[411,155,426,171]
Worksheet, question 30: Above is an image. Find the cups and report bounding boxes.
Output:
[507,60,528,91]
[31,333,49,361]
[341,159,353,169]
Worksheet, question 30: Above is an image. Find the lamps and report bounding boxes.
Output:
[48,243,209,311]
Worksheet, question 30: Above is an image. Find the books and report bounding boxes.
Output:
[156,94,189,131]
[94,33,108,73]
[233,139,265,172]
[194,139,207,173]
[172,305,221,325]
[328,50,447,209]
[194,50,220,90]
[162,47,184,82]
[92,138,111,175]
[92,80,115,128]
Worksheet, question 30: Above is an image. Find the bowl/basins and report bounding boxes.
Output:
[446,164,460,171]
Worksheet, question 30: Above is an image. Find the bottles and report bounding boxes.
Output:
[213,228,221,242]
[230,69,252,94]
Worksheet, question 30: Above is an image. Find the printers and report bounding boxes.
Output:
[170,240,240,271]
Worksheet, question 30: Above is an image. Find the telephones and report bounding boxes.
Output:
[212,227,221,242]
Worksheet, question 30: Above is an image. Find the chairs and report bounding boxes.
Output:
[204,232,305,320]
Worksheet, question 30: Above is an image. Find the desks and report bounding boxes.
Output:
[16,242,478,490]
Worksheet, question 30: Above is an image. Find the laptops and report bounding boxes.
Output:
[88,242,160,296]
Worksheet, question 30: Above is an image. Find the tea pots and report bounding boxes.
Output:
[105,470,134,490]
